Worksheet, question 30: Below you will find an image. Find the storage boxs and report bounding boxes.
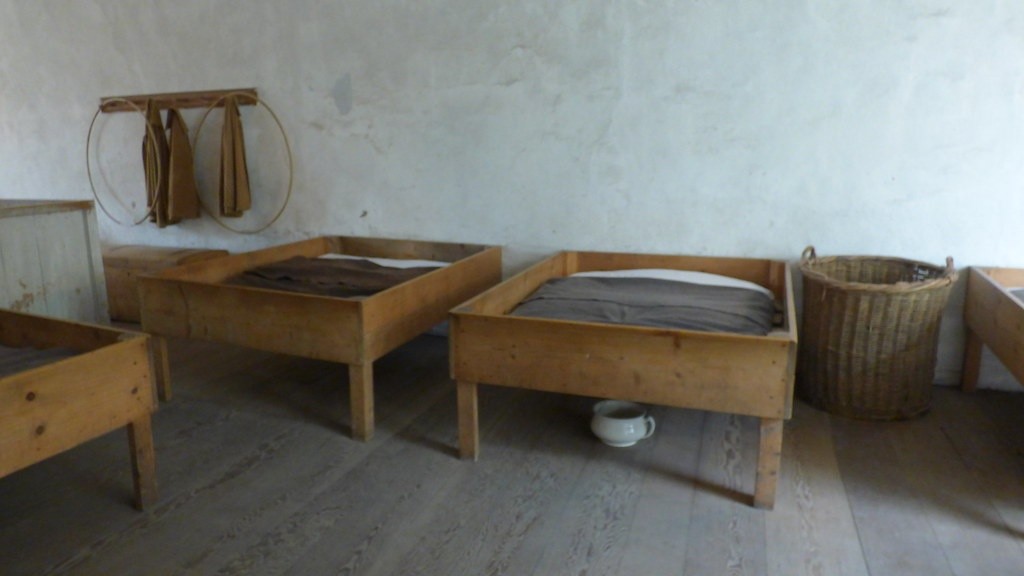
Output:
[798,244,957,420]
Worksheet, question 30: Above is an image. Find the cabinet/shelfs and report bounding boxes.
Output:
[1,197,111,329]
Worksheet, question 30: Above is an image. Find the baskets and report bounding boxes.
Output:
[796,246,960,423]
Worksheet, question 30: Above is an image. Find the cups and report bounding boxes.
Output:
[591,402,657,450]
